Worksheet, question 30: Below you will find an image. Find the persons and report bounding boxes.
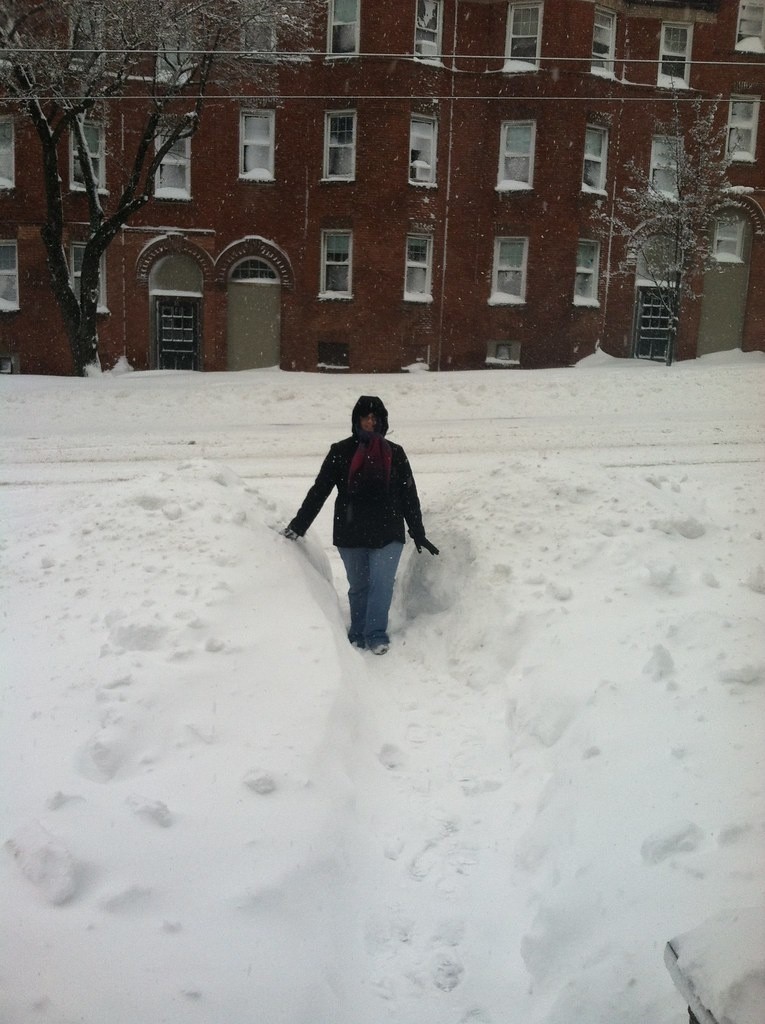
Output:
[281,395,439,654]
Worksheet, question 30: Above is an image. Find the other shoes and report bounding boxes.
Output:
[372,643,388,655]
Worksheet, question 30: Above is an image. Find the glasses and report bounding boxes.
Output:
[361,416,376,422]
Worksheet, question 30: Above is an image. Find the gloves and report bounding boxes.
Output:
[278,528,298,541]
[413,537,439,556]
